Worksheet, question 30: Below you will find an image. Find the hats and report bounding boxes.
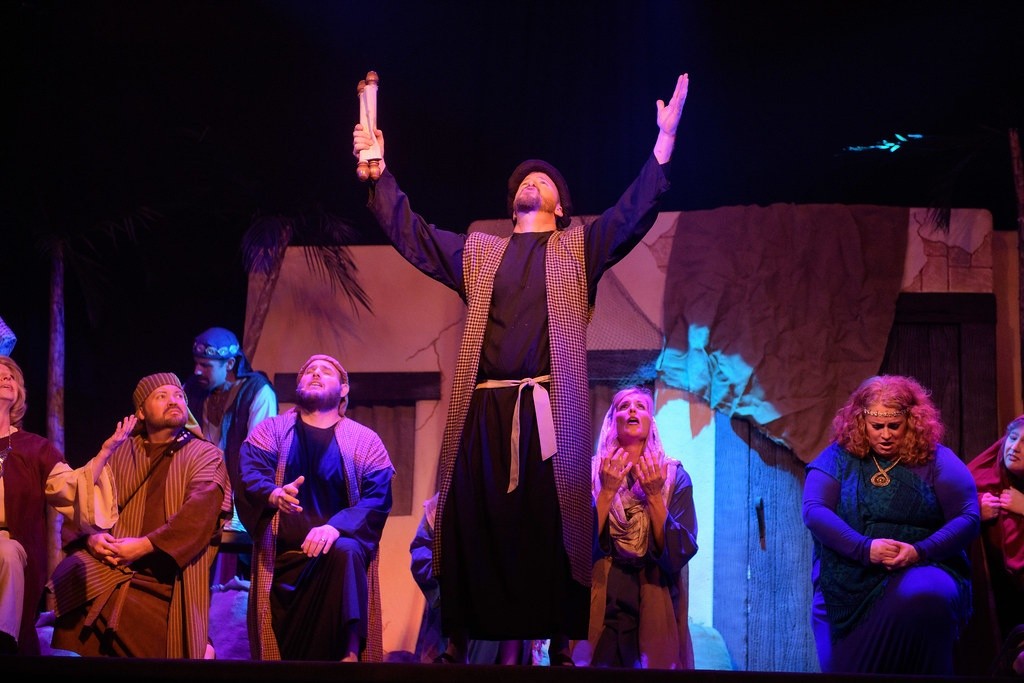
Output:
[508,160,572,231]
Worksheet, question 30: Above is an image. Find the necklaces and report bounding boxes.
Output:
[869,454,902,487]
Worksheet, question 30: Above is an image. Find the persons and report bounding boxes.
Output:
[592,388,698,671]
[408,489,550,667]
[803,376,1005,676]
[238,354,393,663]
[46,372,233,660]
[354,72,688,667]
[964,416,1024,675]
[180,327,280,533]
[0,355,136,655]
